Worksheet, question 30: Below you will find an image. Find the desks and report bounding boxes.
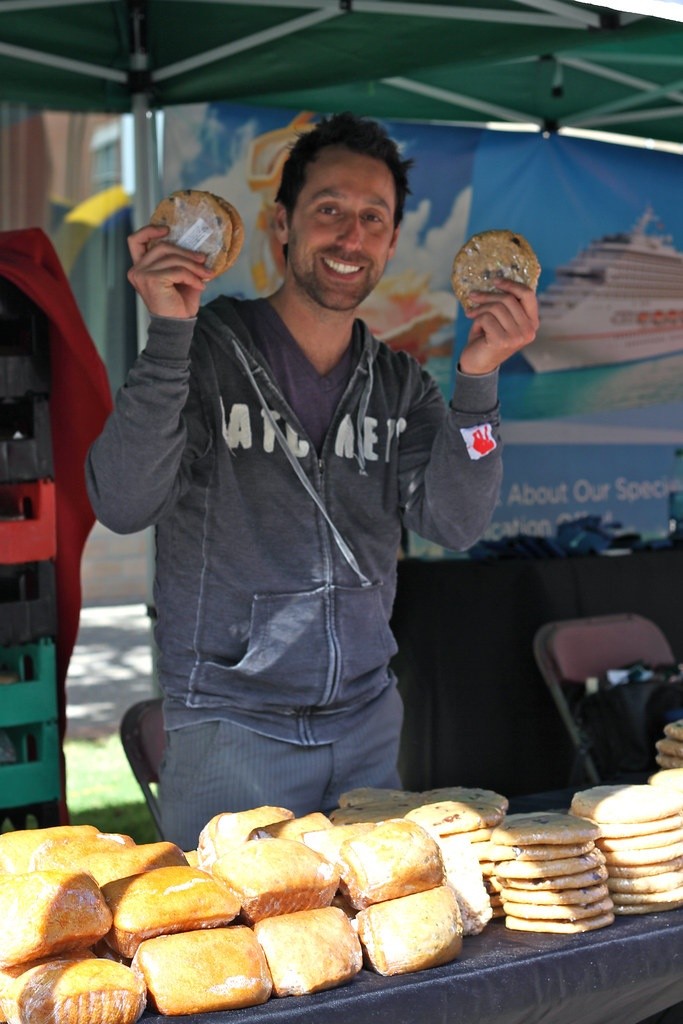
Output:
[133,783,683,1024]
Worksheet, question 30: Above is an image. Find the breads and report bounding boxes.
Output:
[0,721,682,1024]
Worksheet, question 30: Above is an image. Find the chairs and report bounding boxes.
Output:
[531,611,682,787]
[118,695,168,843]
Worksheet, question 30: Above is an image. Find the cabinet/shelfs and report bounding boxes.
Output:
[0,277,62,832]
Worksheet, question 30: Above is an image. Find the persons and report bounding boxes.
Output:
[85,116,538,850]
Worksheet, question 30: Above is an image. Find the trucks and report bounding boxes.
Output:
[93,97,683,563]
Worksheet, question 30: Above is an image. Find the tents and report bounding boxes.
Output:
[2,2,682,345]
